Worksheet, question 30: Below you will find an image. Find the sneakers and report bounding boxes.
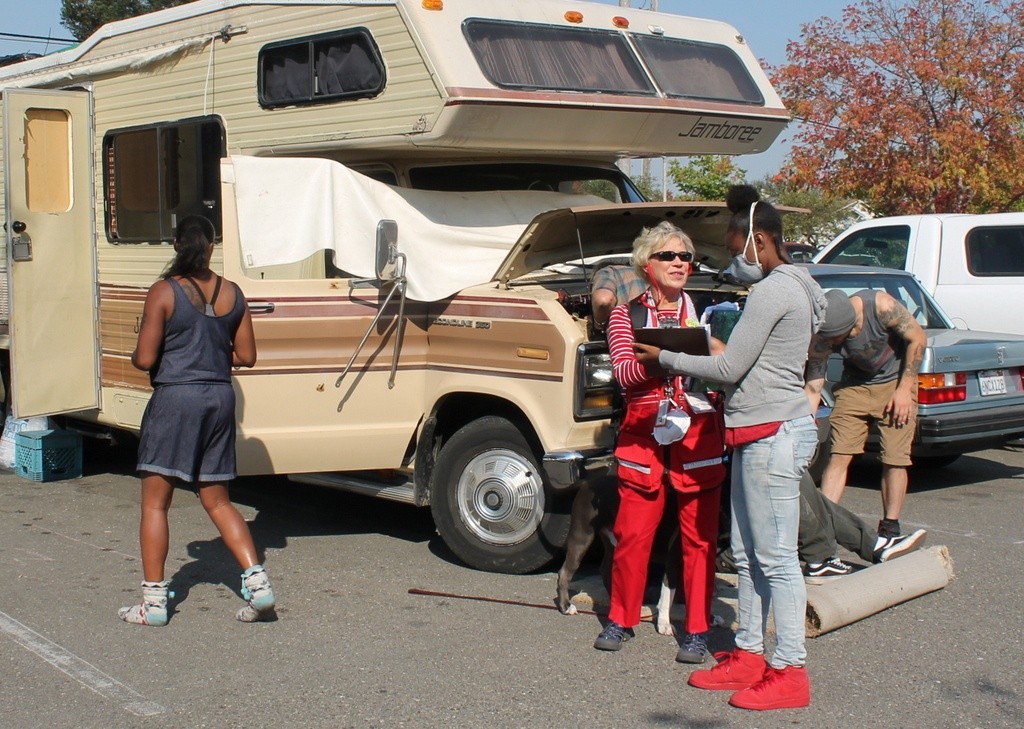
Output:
[803,557,854,578]
[677,631,710,663]
[688,646,767,690]
[729,665,810,710]
[594,621,631,651]
[872,528,927,564]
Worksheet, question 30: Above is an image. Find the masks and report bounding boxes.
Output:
[728,253,764,286]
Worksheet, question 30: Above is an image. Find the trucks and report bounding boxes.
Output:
[1,0,835,575]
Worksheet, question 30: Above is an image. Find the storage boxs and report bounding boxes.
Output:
[14,429,82,482]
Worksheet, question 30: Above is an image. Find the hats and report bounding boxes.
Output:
[817,289,857,336]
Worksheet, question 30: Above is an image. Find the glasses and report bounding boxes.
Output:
[649,251,693,262]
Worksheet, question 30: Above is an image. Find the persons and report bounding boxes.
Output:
[117,212,275,627]
[632,185,828,711]
[592,186,926,712]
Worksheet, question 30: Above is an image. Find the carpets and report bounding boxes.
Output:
[568,545,956,639]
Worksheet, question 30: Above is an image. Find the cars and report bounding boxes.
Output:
[782,244,1024,470]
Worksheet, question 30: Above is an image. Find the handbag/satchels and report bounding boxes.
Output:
[0,415,48,471]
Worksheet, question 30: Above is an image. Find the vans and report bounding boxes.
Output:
[809,212,1024,334]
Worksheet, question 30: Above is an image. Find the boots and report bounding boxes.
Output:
[117,579,175,627]
[234,564,275,621]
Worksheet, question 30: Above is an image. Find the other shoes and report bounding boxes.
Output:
[879,518,900,538]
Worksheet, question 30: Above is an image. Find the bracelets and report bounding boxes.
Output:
[594,318,608,326]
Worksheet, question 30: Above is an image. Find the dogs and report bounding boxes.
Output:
[556,456,730,637]
